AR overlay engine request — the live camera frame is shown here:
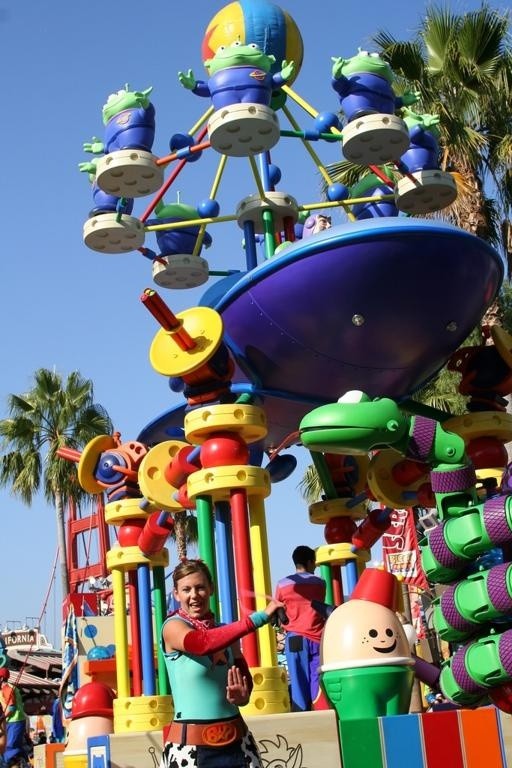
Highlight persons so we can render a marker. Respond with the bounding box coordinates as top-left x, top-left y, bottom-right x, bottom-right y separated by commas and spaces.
0, 668, 30, 768
276, 546, 326, 711
160, 560, 287, 768
